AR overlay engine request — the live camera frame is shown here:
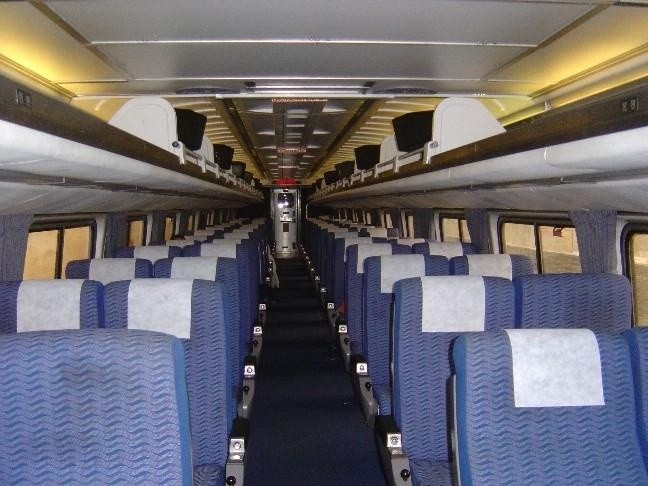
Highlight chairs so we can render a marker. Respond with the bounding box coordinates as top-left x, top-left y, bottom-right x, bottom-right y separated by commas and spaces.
447, 325, 647, 486
392, 275, 513, 486
518, 272, 636, 332
0, 279, 101, 334
623, 324, 647, 457
0, 328, 194, 486
65, 258, 153, 286
